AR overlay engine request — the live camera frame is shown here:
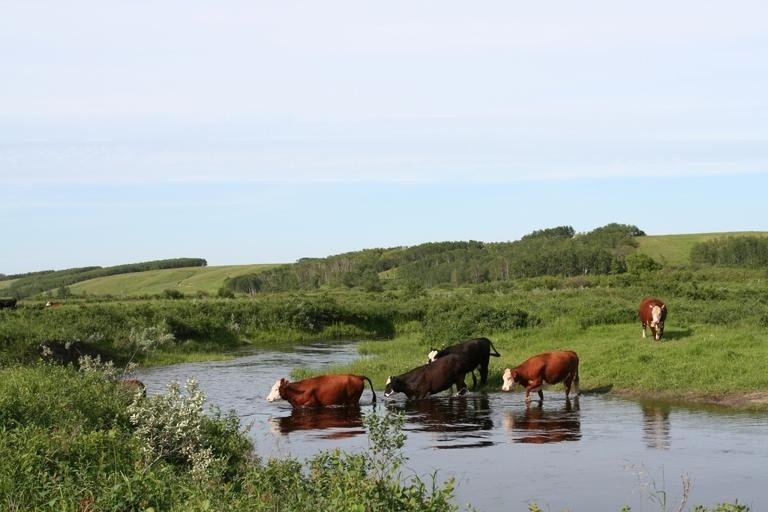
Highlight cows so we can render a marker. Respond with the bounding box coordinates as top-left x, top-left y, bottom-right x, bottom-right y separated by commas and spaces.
638, 296, 667, 341
266, 373, 376, 410
121, 379, 146, 399
501, 350, 579, 401
268, 410, 367, 440
384, 353, 477, 401
427, 337, 500, 385
